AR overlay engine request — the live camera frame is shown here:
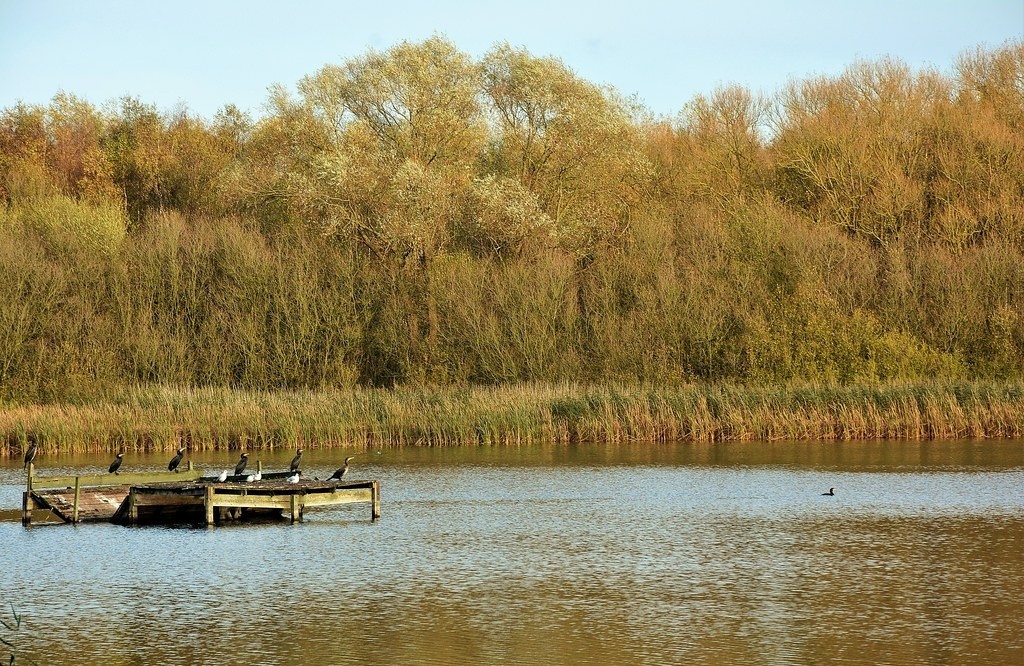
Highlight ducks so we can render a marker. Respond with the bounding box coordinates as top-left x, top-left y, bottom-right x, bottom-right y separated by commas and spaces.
109, 447, 355, 484
820, 487, 836, 496
